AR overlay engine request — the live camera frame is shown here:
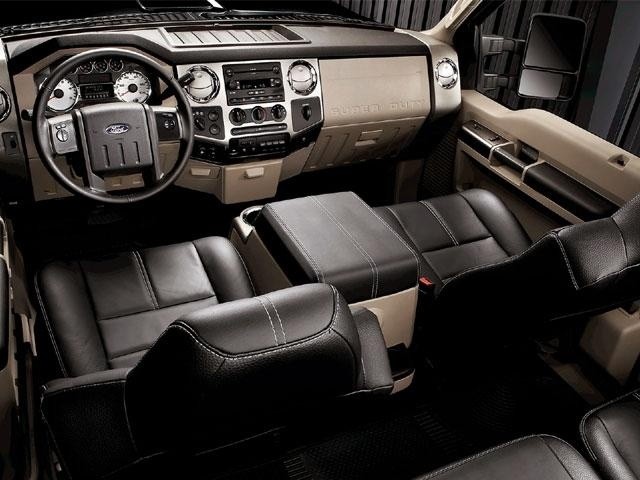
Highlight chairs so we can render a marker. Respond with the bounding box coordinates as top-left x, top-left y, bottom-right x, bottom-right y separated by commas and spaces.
368, 171, 640, 437
574, 383, 640, 480
410, 430, 603, 480
26, 227, 400, 480
